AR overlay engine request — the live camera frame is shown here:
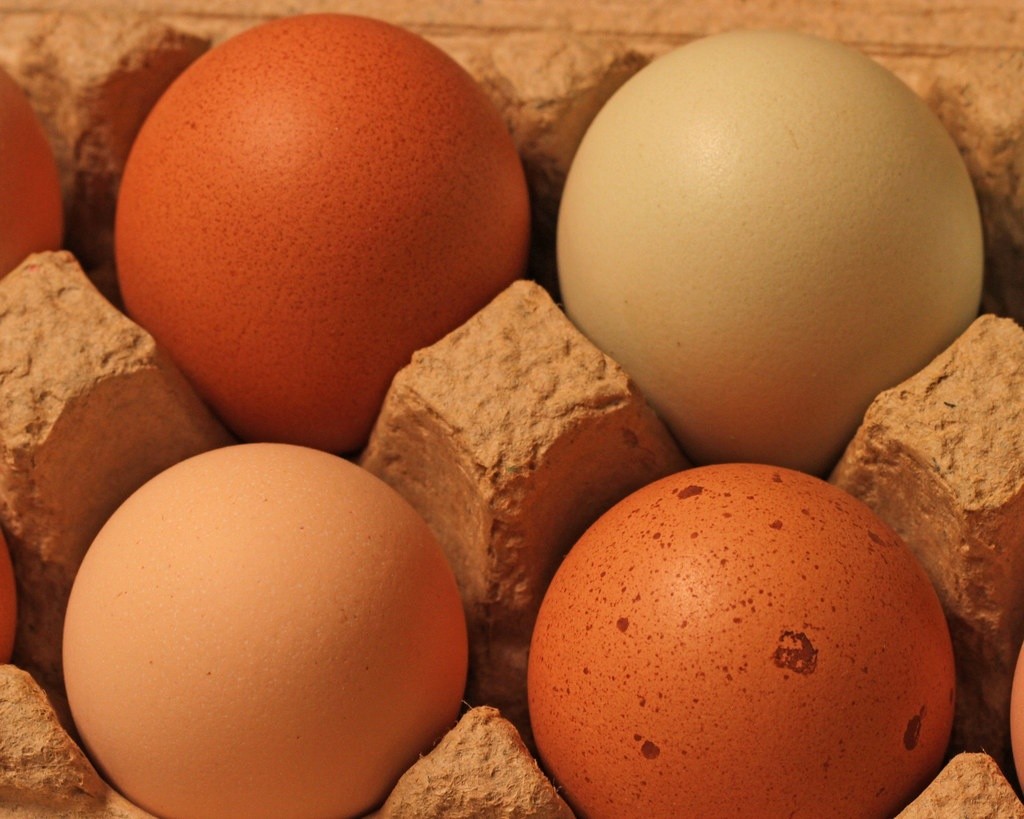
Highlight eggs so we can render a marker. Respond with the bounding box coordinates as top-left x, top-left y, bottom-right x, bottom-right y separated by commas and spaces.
0, 13, 1024, 819
